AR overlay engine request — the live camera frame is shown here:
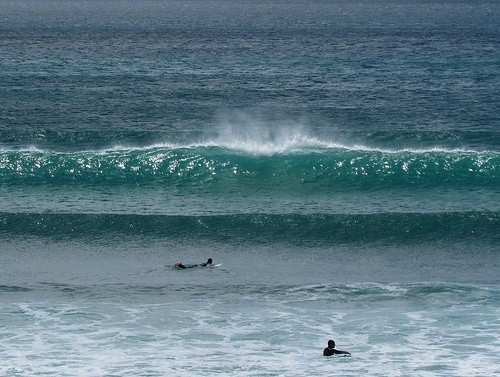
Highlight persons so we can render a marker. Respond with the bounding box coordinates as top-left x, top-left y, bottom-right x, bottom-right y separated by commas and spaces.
175, 258, 213, 270
323, 340, 350, 356
